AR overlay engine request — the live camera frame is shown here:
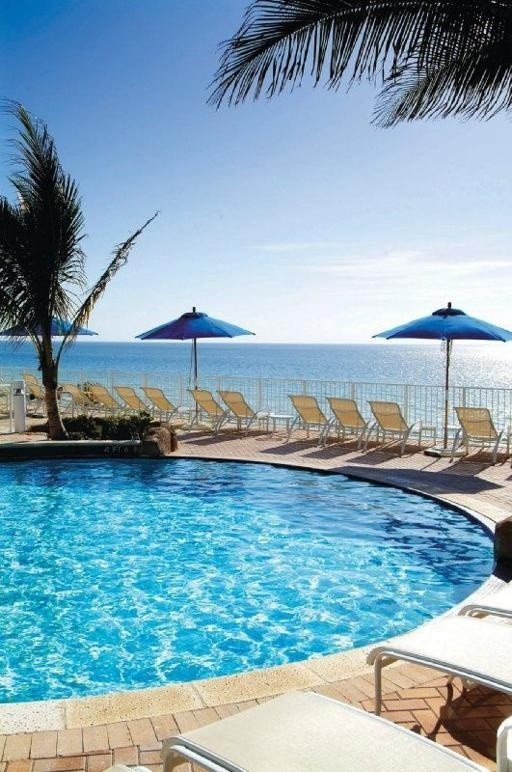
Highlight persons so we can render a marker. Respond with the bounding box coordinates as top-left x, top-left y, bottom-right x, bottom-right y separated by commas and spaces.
0, 311, 99, 337
374, 298, 512, 449
136, 304, 256, 414
